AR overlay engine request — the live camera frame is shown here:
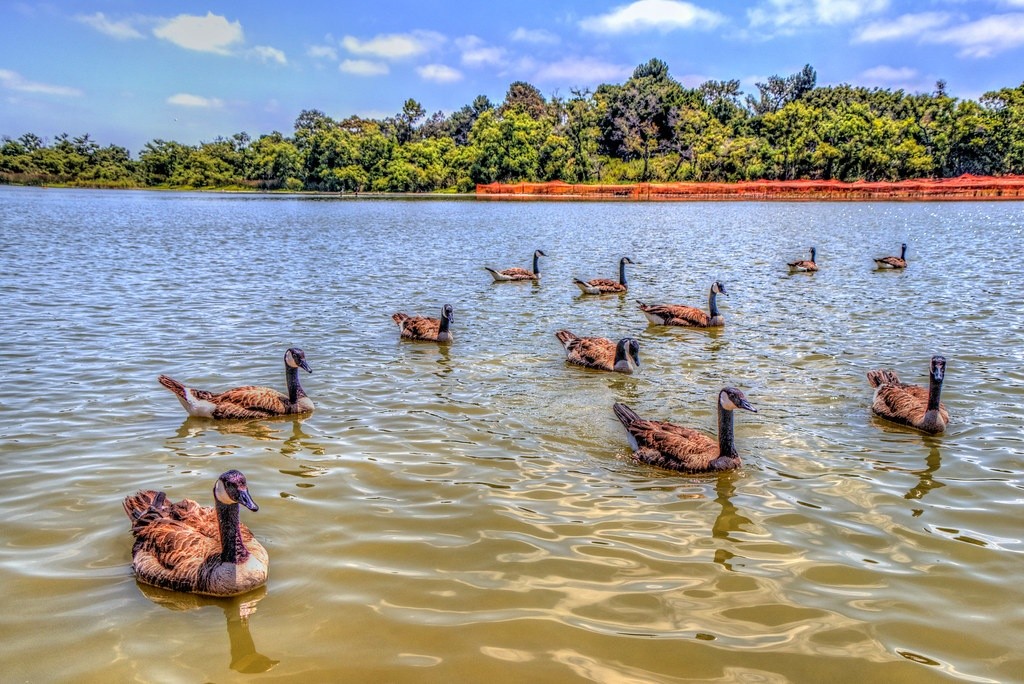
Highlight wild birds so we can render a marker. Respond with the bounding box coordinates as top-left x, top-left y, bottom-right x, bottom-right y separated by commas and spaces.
484, 249, 547, 282
121, 469, 270, 598
872, 242, 908, 269
787, 247, 819, 272
867, 355, 949, 435
392, 304, 454, 346
572, 257, 635, 297
158, 348, 315, 422
613, 387, 758, 482
555, 330, 640, 375
636, 281, 730, 329
